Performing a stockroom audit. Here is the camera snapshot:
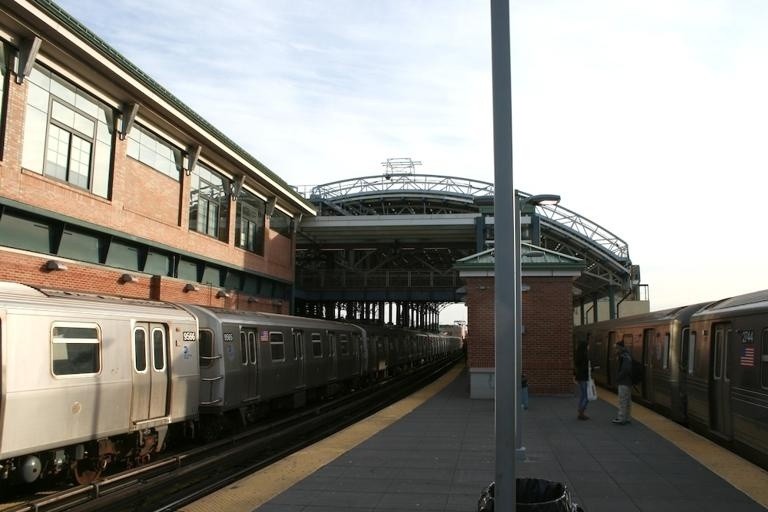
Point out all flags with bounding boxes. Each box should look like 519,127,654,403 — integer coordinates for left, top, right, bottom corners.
260,330,270,342
739,343,757,368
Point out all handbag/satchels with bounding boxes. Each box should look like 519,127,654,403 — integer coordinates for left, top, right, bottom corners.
587,379,597,402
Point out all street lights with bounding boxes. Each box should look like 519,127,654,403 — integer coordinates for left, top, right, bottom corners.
471,189,563,461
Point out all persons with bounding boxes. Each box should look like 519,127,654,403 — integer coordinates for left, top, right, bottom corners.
611,340,633,424
574,341,594,420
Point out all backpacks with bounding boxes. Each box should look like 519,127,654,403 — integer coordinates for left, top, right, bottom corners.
632,360,643,385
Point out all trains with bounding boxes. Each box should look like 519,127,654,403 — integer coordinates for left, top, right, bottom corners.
573,289,768,469
0,281,465,489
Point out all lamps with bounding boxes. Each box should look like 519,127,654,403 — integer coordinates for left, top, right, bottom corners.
248,297,258,302
186,283,200,292
457,287,467,294
219,290,230,298
41,259,68,272
122,273,141,283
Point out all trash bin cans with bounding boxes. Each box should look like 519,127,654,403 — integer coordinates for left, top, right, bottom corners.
520,376,529,410
478,478,578,511
